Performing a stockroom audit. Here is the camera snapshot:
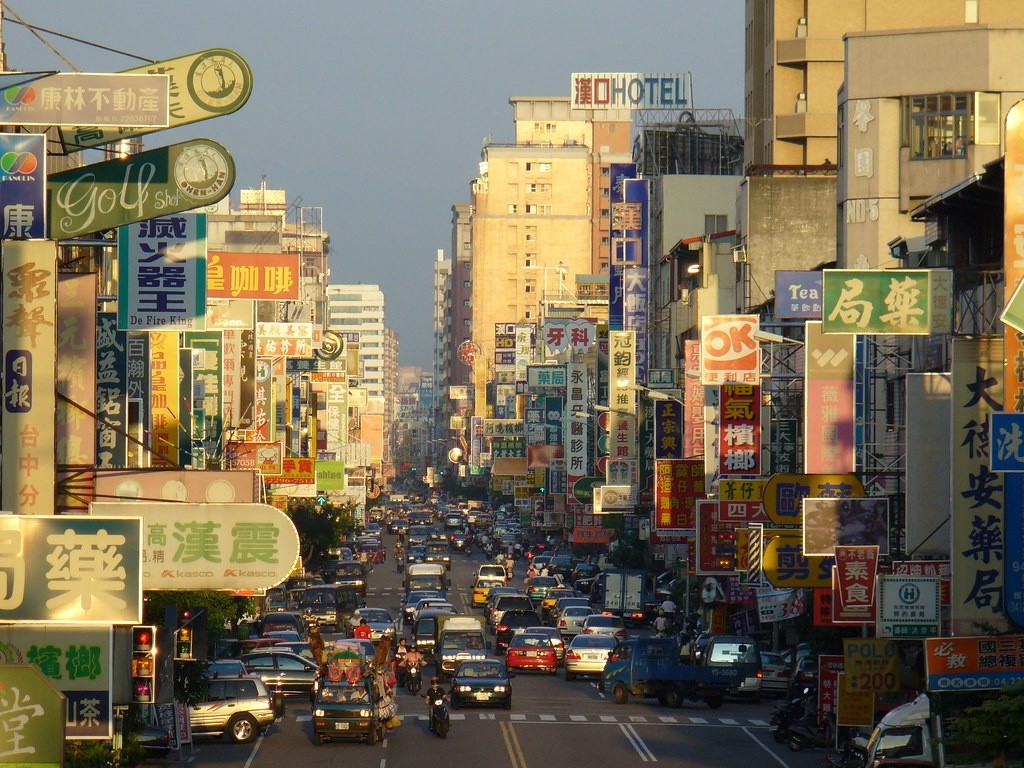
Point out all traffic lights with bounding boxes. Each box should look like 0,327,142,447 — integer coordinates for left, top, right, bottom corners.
529,486,545,493
289,495,327,506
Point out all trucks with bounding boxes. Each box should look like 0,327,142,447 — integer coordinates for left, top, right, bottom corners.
433,615,491,685
311,674,387,746
600,636,746,711
864,693,1024,768
599,567,647,630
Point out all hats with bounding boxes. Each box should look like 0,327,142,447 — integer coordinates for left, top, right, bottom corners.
659,610,664,615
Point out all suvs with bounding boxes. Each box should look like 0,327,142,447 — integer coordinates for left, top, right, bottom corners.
187,672,285,743
493,609,543,657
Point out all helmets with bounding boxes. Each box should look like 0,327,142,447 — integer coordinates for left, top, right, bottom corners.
409,643,416,648
431,677,439,684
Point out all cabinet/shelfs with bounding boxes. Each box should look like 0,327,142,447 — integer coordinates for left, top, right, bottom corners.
131,625,155,703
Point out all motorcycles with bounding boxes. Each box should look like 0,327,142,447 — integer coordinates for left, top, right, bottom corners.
420,688,453,739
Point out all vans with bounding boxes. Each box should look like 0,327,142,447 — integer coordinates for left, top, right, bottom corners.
702,635,764,705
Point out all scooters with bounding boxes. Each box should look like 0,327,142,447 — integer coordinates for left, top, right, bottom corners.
401,655,426,697
389,647,407,688
769,686,851,753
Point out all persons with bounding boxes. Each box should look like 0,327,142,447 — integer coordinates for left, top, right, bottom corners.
394,638,412,659
355,549,375,573
584,554,591,563
350,610,363,626
351,686,368,702
465,531,493,559
495,542,522,576
394,526,406,572
591,575,599,592
653,596,677,634
355,619,372,639
527,562,557,578
404,647,427,682
487,666,501,678
699,576,724,603
467,636,483,648
303,607,317,620
680,609,703,656
425,676,450,730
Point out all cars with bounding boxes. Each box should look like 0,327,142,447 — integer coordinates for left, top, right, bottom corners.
523,627,565,668
235,651,320,698
216,494,603,664
553,605,595,635
502,633,557,678
645,589,673,624
576,615,627,644
199,659,251,676
759,642,817,701
127,723,172,759
563,633,620,682
447,659,516,711
